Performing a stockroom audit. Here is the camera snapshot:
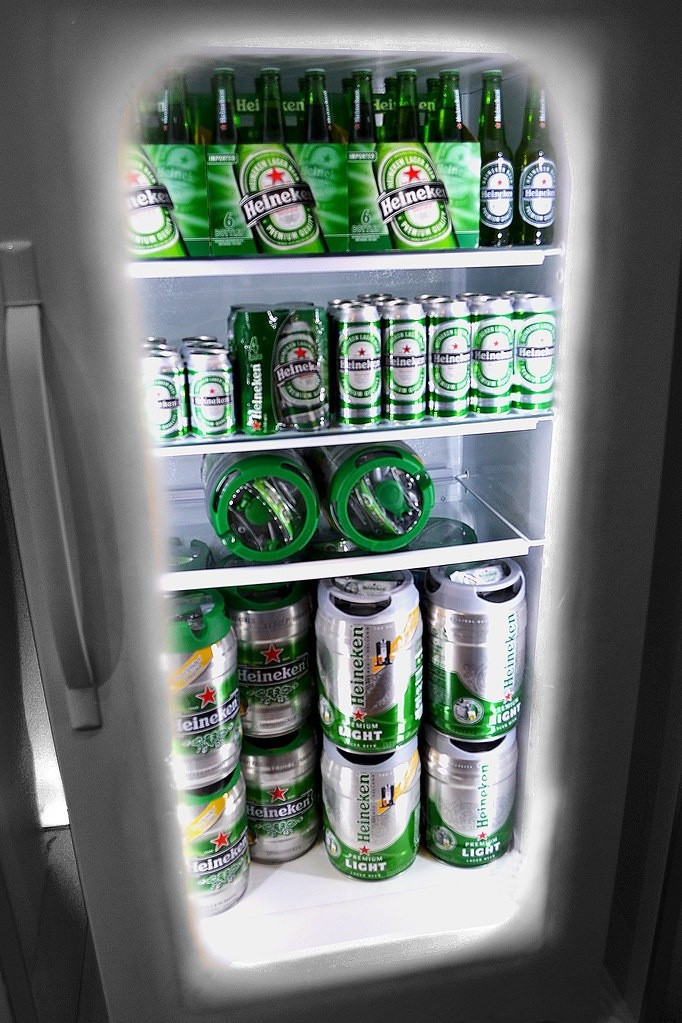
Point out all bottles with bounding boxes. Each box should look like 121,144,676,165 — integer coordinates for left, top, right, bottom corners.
372,144,460,250
127,66,463,143
232,143,330,255
478,69,516,247
515,73,556,247
125,144,190,261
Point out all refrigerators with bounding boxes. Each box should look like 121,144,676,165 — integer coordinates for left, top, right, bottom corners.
1,0,682,1023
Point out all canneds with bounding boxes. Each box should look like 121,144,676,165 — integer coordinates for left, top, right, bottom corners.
139,286,557,445
157,443,527,916
